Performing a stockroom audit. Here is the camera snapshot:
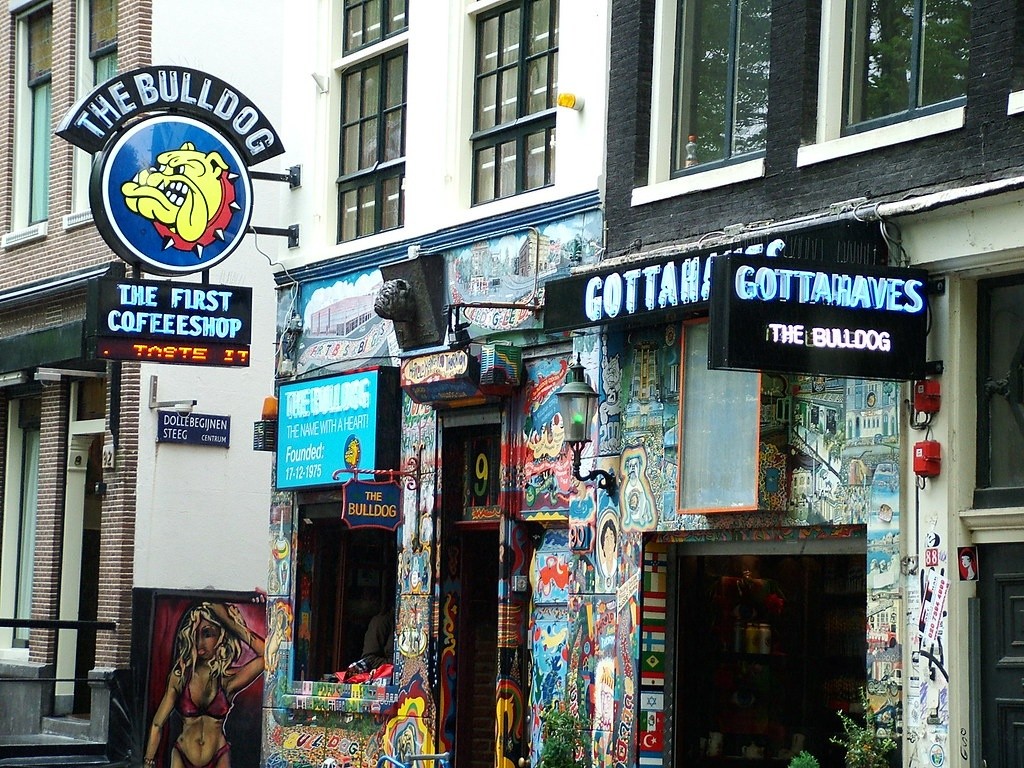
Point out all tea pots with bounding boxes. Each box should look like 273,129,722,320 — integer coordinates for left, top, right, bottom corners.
742,741,764,759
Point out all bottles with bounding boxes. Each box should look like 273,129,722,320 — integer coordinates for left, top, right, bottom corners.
745,623,759,653
760,623,771,653
730,617,744,651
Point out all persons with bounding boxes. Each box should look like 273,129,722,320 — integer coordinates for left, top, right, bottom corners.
361,586,396,670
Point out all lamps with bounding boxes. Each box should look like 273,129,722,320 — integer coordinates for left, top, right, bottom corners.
554,352,616,498
558,92,585,112
253,395,277,452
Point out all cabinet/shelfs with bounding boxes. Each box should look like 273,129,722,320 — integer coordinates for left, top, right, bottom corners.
684,555,815,768
330,531,384,672
815,556,866,768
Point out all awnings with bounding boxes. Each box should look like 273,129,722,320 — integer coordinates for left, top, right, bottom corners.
544,219,888,332
